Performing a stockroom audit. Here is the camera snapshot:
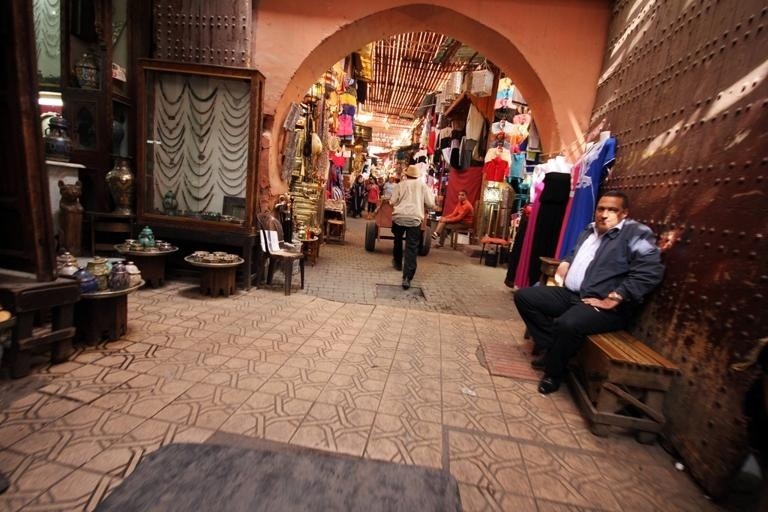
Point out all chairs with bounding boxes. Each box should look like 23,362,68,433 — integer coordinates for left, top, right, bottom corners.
451,200,482,250
479,202,512,265
256,214,307,297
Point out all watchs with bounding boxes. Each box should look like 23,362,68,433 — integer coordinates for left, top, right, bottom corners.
609,291,622,301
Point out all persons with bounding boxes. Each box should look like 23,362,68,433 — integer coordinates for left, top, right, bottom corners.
349,175,367,218
366,176,381,221
554,129,620,263
383,177,396,199
512,190,664,391
502,154,573,289
388,165,440,290
430,189,474,249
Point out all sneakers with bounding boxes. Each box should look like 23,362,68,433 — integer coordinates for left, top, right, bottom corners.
392,258,402,272
401,278,410,287
432,233,439,239
434,243,444,250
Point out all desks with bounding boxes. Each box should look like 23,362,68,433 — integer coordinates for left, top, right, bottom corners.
59,264,146,345
115,241,179,288
184,250,245,296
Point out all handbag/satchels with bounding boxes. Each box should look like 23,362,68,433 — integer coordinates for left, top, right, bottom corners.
309,116,324,157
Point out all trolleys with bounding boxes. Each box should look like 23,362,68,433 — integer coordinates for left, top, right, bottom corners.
364,196,434,255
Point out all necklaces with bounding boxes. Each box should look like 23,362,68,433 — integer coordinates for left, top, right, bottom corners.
156,78,183,213
221,84,249,197
183,82,219,212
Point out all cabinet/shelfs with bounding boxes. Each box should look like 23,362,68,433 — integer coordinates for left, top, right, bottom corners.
148,71,266,255
61,0,137,258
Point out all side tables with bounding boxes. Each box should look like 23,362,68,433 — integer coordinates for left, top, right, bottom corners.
295,232,320,266
326,220,345,246
537,255,562,288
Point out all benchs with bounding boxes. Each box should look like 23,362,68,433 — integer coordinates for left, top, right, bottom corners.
539,281,681,448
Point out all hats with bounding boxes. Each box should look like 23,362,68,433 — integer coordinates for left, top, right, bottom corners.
403,166,422,179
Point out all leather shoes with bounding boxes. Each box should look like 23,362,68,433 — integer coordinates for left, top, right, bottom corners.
537,374,561,396
529,356,547,366
